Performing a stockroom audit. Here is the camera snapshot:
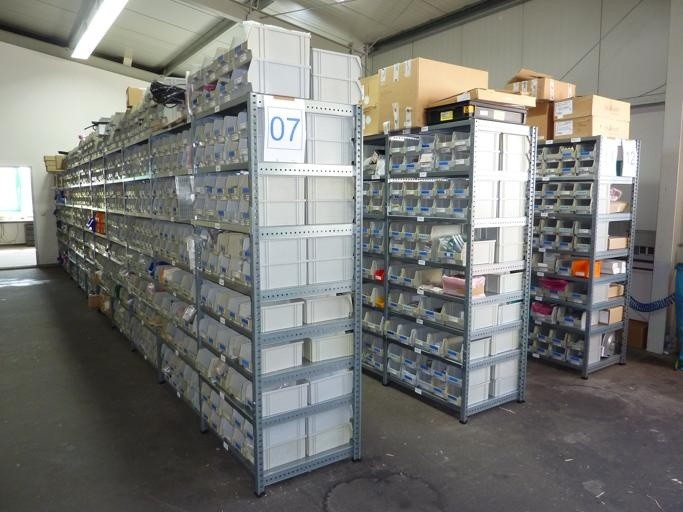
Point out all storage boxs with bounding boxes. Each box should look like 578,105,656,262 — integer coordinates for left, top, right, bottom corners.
627,319,648,349
377,56,490,134
357,74,382,136
504,68,630,140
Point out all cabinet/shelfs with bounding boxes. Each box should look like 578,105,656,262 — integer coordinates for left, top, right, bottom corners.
55,20,364,498
526,133,641,380
361,118,539,425
